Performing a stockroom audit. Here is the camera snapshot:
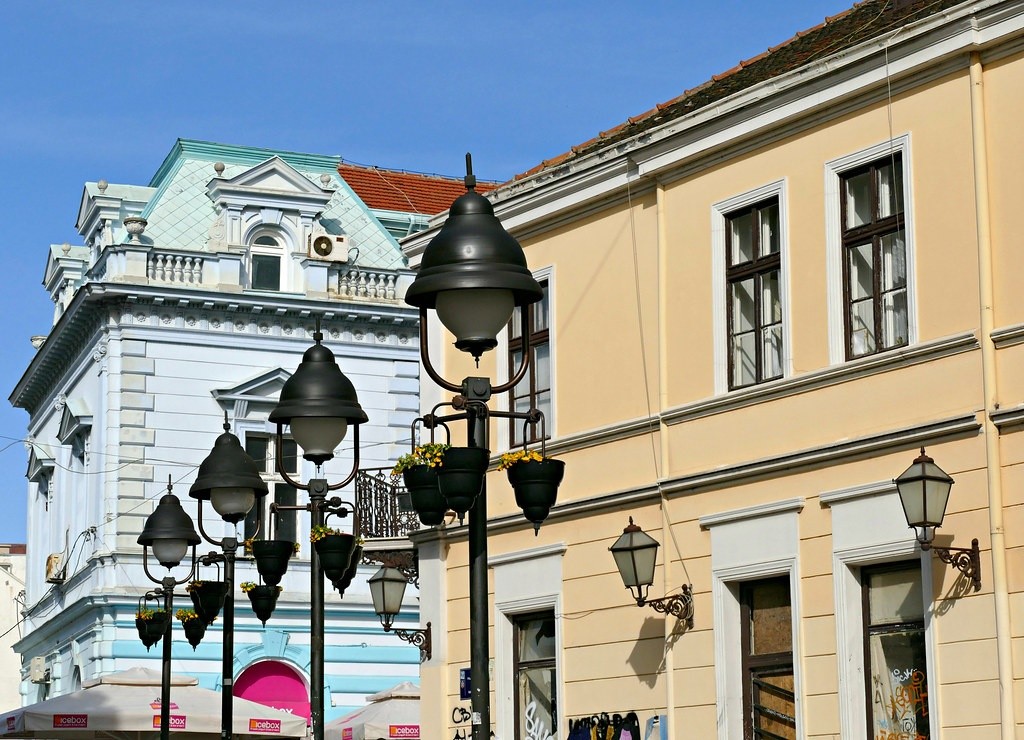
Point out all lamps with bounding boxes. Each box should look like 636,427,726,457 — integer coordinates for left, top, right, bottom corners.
609,516,697,630
893,444,982,593
366,560,432,661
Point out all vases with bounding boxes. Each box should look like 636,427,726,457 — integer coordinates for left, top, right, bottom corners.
247,585,283,628
506,457,566,537
336,545,362,599
402,465,447,527
252,541,295,597
190,590,210,631
314,534,356,591
195,581,229,627
149,611,171,647
436,446,491,527
136,618,156,652
182,618,208,652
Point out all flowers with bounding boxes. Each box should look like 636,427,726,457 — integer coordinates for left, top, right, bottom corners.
416,444,452,467
157,608,167,612
241,581,256,593
175,608,198,623
245,538,255,554
293,542,301,552
309,524,340,543
497,449,551,471
188,580,203,587
392,452,419,476
356,538,364,547
135,606,154,620
186,585,195,593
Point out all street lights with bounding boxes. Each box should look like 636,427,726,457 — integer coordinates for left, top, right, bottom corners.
190,409,285,740
136,468,210,740
400,152,569,740
268,313,379,740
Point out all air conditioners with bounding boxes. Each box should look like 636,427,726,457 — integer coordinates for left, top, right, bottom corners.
30,656,47,682
45,553,64,584
308,232,349,263
